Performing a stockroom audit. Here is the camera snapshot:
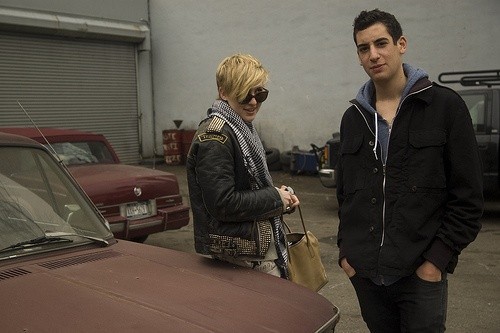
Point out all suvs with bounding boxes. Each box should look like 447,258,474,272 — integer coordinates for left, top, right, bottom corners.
320,69,500,202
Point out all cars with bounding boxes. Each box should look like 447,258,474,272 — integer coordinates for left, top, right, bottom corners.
0,126,191,243
0,131,341,333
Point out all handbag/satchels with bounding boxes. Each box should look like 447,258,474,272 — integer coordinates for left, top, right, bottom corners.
283,186,329,293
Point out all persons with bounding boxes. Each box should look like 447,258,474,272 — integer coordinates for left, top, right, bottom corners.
188,54,300,278
336,9,483,333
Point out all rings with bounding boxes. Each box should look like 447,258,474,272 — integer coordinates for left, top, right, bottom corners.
287,206,290,209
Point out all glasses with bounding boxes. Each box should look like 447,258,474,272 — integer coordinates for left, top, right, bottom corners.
237,88,269,104
